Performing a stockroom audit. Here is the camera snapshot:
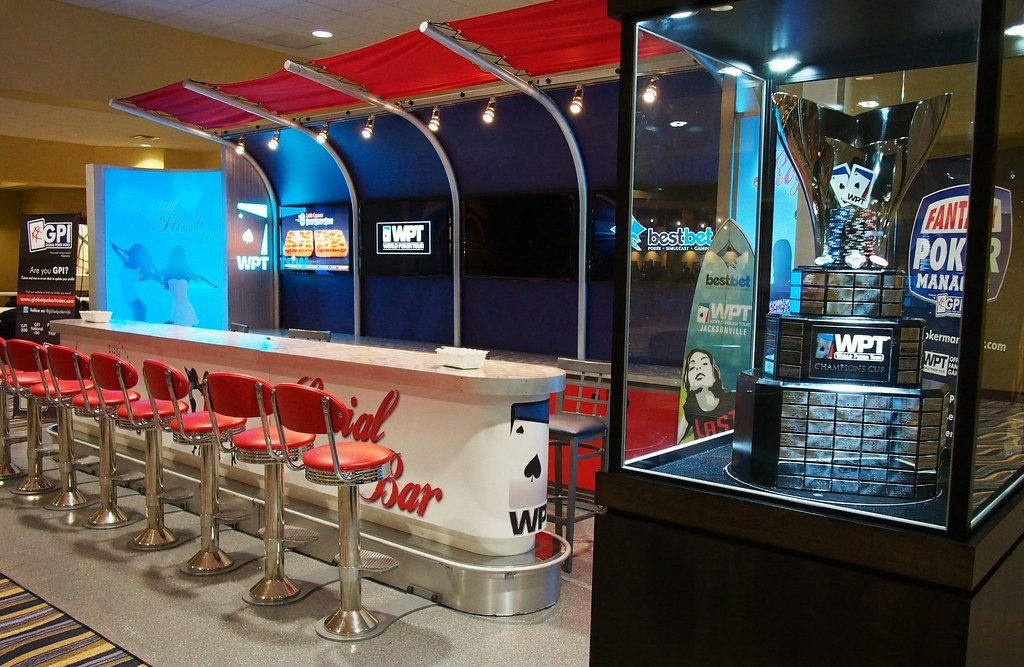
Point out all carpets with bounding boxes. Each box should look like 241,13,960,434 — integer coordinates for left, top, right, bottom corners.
0,572,149,667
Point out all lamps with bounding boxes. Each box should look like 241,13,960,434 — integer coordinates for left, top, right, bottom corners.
267,128,280,149
361,114,375,138
236,134,246,154
643,74,660,102
429,107,440,132
483,98,496,122
317,122,328,143
570,84,584,112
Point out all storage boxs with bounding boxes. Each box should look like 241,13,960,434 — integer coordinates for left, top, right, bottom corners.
435,345,489,369
79,310,113,323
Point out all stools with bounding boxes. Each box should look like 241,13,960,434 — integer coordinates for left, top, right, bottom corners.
0,338,400,642
547,357,611,573
